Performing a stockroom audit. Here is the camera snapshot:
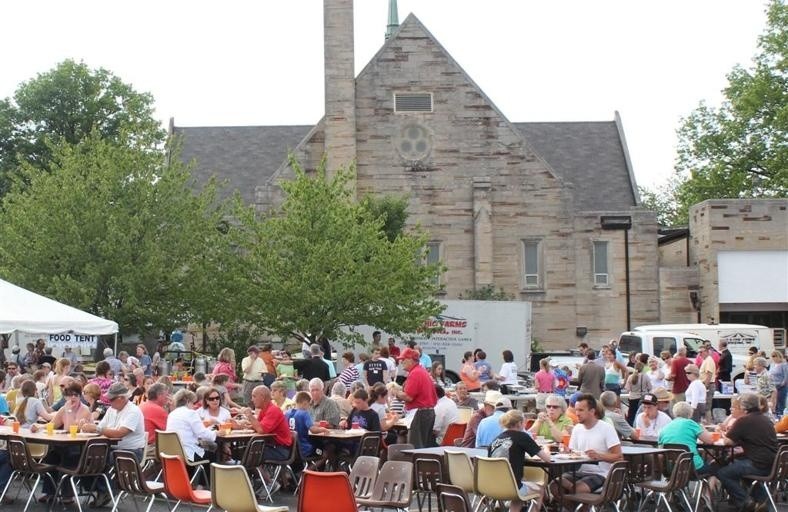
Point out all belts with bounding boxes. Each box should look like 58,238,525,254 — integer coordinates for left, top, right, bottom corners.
418,407,433,410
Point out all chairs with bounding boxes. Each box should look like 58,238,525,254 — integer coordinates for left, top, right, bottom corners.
160,452,214,512
235,430,304,495
111,449,171,512
413,453,445,512
356,460,414,512
210,463,291,512
50,438,119,512
444,451,488,511
203,435,277,505
661,444,711,511
0,436,65,512
8,442,48,502
436,485,471,512
154,430,209,493
100,431,148,503
473,455,541,512
298,470,359,512
388,445,422,512
348,456,380,512
640,452,695,512
726,447,783,512
440,423,467,445
343,432,381,468
563,461,629,512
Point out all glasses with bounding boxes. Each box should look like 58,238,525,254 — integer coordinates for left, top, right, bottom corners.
67,393,79,397
206,396,221,401
707,344,711,346
8,368,16,370
546,405,560,409
749,353,754,354
700,350,706,353
686,372,692,374
60,385,65,387
123,378,130,381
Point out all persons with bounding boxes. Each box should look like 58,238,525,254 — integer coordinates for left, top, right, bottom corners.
231,385,293,499
258,344,277,387
138,383,169,455
366,331,388,358
40,362,54,383
292,378,309,402
1,362,20,393
378,347,396,385
40,347,56,364
714,392,778,512
60,344,78,367
148,375,173,414
755,395,775,428
657,401,717,512
33,339,45,360
604,348,629,413
95,425,103,434
480,381,513,409
633,387,671,427
196,389,232,463
577,343,589,364
596,344,611,392
699,345,716,424
719,394,768,503
31,381,96,503
534,358,555,413
275,351,298,378
460,351,483,392
550,394,624,512
52,358,74,404
136,344,152,375
596,390,641,481
355,352,368,379
118,351,144,386
83,383,107,421
211,347,240,400
641,356,665,393
454,390,502,470
22,343,37,365
489,350,518,394
561,365,572,376
81,382,146,508
717,339,732,416
576,348,605,400
102,348,128,382
624,363,652,427
32,370,49,401
487,409,551,512
285,392,329,472
753,357,778,414
395,341,417,385
471,351,492,393
431,384,460,447
330,382,354,420
368,386,406,451
473,349,506,381
386,348,438,449
330,353,360,398
270,380,294,416
49,378,89,412
133,368,146,405
672,343,688,360
631,393,672,477
317,345,338,379
210,373,245,411
388,338,400,365
151,352,162,376
475,398,547,462
640,354,651,373
368,381,392,412
0,371,17,504
193,372,211,405
695,340,720,420
5,345,28,372
324,339,338,361
771,350,788,420
166,389,218,490
270,343,330,380
241,345,269,409
172,357,187,371
684,364,707,422
363,347,388,393
430,361,448,388
307,377,340,440
325,389,381,475
124,373,142,406
13,381,57,430
453,380,478,412
757,350,766,358
414,345,432,373
627,352,637,368
67,365,89,382
136,377,154,405
610,340,625,365
528,396,574,443
549,360,569,397
744,347,759,384
347,381,364,408
665,345,694,420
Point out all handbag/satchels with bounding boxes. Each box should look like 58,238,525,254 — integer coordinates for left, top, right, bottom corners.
638,374,645,405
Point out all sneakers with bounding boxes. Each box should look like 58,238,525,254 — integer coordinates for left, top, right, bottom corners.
255,480,281,499
699,494,712,511
0,496,14,506
39,495,58,502
59,497,75,504
88,491,116,508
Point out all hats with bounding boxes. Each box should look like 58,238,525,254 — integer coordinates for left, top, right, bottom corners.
104,348,113,356
649,387,674,401
549,360,559,366
641,393,657,406
396,348,420,360
12,345,20,352
103,383,128,400
495,398,512,408
248,345,262,353
484,391,502,406
41,362,51,369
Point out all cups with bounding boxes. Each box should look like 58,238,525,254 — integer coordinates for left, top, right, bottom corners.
536,435,546,446
635,429,640,438
219,424,226,434
12,422,20,434
561,436,571,448
713,432,722,440
188,376,193,382
225,423,232,435
181,376,187,382
70,425,77,439
47,423,54,436
352,422,359,429
319,420,327,432
173,376,177,381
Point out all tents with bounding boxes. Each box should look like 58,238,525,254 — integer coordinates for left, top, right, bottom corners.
0,278,120,358
